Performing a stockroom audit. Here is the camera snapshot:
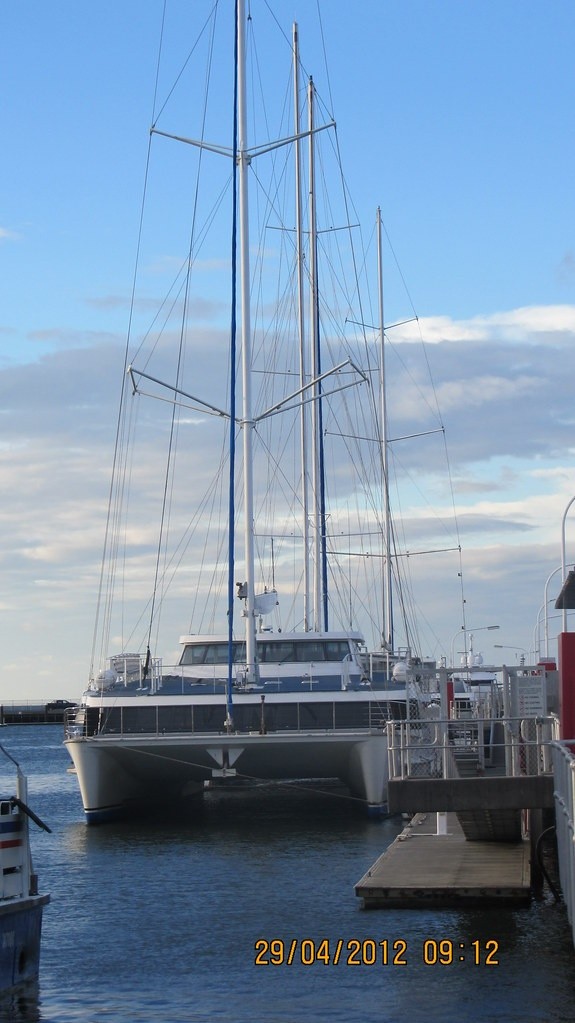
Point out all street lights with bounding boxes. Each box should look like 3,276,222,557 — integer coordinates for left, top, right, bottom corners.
495,644,530,666
450,625,501,670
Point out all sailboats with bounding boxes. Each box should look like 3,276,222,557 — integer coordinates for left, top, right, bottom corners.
58,2,447,831
